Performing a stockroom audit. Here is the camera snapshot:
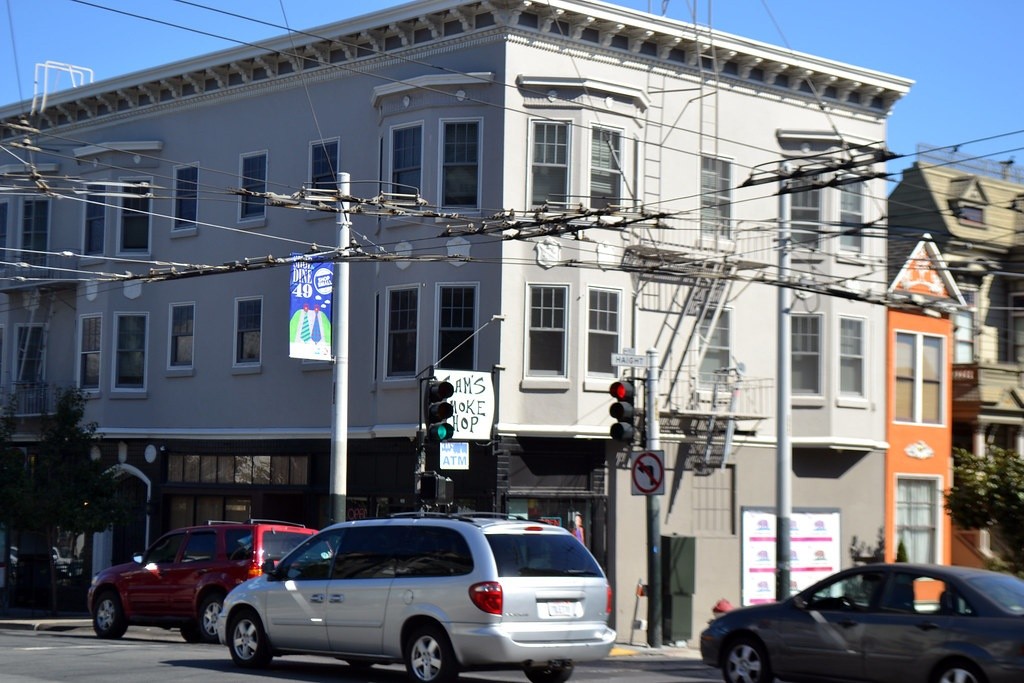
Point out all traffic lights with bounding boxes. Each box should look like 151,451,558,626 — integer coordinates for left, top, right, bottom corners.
423,474,453,506
424,376,458,444
609,377,636,443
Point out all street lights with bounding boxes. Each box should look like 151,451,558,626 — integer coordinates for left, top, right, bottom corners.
123,464,153,551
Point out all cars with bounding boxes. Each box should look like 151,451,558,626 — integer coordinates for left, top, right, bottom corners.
699,559,1024,682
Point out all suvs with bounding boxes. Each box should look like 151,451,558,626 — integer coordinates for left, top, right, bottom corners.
85,516,318,647
215,511,618,682
